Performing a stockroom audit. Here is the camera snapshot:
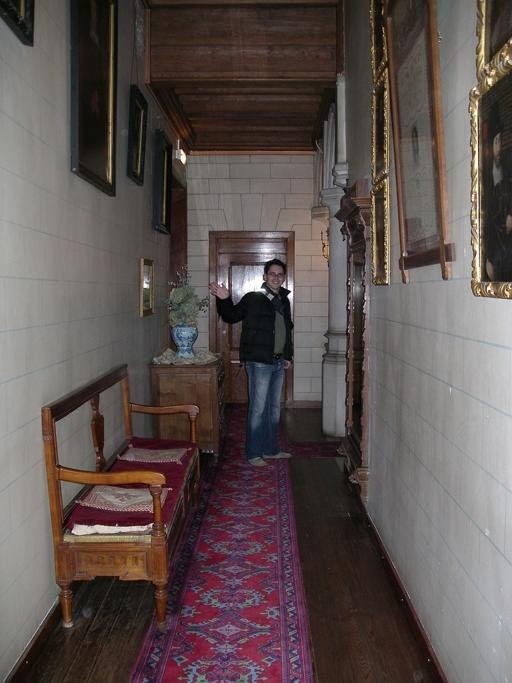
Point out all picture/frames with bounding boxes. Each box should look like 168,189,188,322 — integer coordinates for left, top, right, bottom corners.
389,2,457,290
70,0,118,197
0,0,34,46
154,131,173,234
127,83,148,186
471,4,511,291
140,258,155,318
370,4,388,288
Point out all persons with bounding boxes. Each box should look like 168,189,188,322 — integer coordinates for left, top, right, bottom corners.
481,101,512,282
208,256,295,468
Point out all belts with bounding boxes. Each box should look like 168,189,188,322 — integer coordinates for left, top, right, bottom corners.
272,352,284,360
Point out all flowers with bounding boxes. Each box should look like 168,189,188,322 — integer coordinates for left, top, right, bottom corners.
166,267,208,326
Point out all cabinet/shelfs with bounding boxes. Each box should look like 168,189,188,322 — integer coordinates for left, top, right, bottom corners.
146,347,226,467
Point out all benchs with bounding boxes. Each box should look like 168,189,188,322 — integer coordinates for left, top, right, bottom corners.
41,361,199,631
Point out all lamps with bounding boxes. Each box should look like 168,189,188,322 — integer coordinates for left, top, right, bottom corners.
171,326,198,358
